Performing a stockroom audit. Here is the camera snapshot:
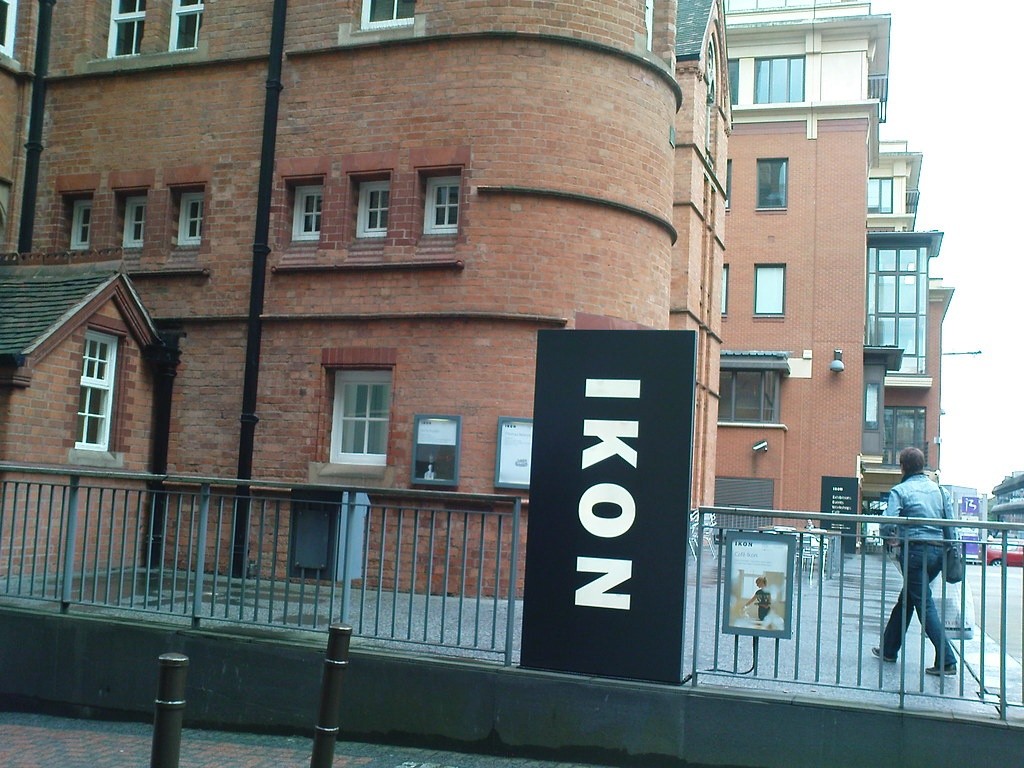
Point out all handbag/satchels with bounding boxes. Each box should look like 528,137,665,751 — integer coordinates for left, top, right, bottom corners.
947,547,962,584
920,571,974,639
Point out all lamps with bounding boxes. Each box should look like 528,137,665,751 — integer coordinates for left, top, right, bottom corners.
830,350,844,372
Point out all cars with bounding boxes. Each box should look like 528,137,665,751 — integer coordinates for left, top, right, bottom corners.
978,537,1024,569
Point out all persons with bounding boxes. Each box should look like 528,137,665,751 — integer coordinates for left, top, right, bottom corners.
870,447,957,675
804,519,816,529
746,577,771,621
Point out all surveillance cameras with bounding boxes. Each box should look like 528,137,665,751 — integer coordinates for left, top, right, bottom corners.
752,441,767,453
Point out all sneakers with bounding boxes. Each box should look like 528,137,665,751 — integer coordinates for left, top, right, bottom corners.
926,663,956,675
872,648,897,662
